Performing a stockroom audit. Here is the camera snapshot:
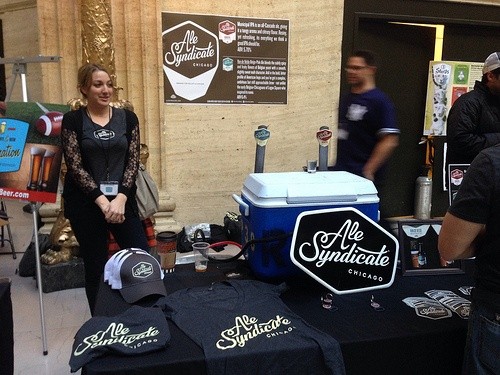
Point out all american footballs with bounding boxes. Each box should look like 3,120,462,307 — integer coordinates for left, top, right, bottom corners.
35,111,64,137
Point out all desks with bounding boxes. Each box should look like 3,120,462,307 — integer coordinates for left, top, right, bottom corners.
78,250,476,374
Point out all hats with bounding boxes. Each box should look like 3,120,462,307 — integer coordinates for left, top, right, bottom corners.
104,247,167,303
484,52,500,74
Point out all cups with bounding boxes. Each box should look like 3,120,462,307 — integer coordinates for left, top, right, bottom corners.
42,151,56,187
27,147,46,188
156,231,177,273
192,243,209,272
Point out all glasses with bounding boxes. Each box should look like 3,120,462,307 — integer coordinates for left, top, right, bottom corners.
344,65,367,70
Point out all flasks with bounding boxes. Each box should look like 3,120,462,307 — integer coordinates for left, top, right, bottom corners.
414,177,432,220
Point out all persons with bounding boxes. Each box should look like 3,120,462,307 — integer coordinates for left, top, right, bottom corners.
60,62,151,317
438,143,500,375
446,50,500,168
332,50,401,181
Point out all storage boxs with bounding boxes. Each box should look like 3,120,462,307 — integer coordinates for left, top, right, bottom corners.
232,171,381,276
